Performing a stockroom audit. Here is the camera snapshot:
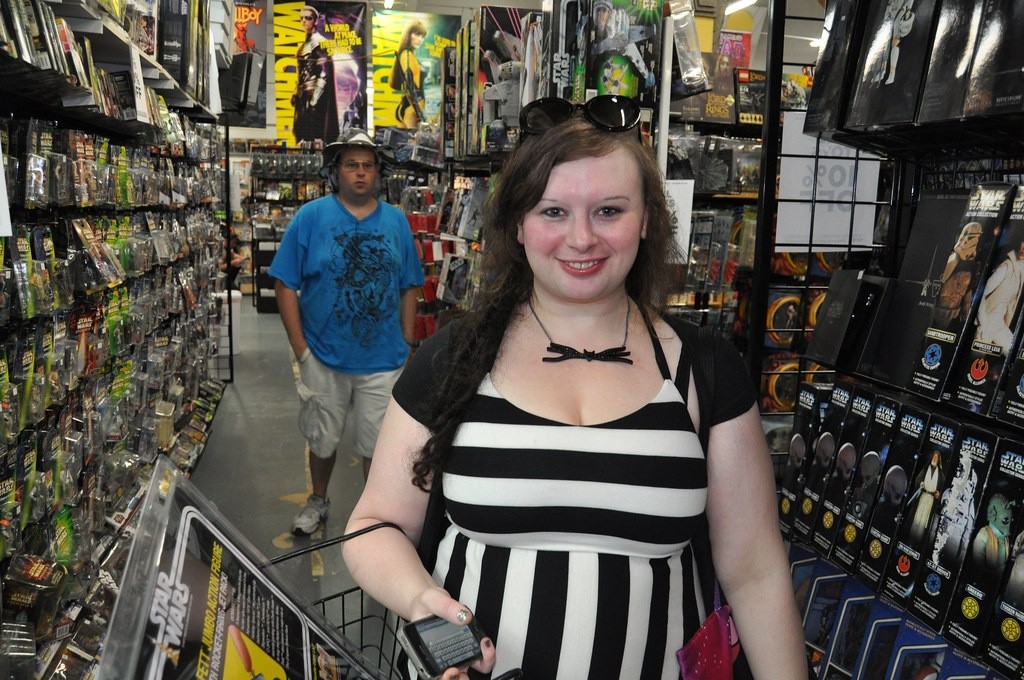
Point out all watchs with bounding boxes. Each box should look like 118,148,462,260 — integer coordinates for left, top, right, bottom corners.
407,339,421,348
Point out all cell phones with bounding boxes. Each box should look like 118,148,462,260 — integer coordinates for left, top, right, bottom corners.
395,605,486,680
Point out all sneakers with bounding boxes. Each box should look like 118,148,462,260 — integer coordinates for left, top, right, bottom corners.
290,493,328,536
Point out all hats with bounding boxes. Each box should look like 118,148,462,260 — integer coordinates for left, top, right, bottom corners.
322,127,399,193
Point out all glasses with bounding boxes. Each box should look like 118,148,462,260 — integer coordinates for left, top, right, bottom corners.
300,16,313,21
335,160,381,173
519,93,643,144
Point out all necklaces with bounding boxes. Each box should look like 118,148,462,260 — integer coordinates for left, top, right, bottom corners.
527,292,634,365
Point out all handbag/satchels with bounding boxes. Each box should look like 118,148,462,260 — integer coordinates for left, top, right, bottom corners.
674,324,756,680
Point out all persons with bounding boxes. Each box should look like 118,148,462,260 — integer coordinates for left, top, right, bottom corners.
714,53,732,92
388,20,428,129
973,236,1024,357
591,0,613,46
341,94,808,680
267,129,426,535
290,6,341,144
905,450,947,540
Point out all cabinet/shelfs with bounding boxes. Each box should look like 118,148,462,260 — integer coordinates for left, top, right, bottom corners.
1,2,1024,680
